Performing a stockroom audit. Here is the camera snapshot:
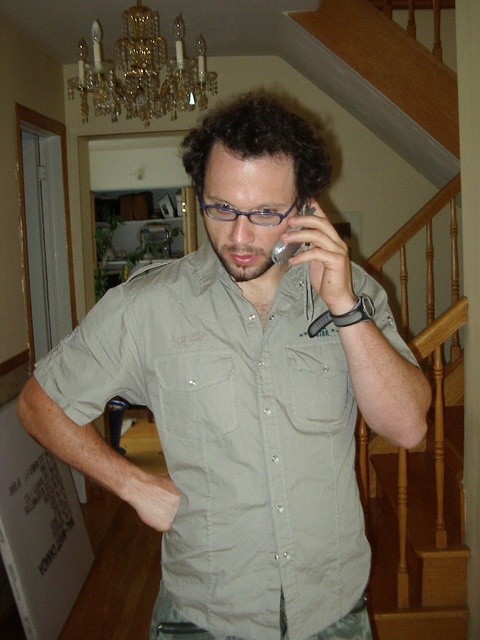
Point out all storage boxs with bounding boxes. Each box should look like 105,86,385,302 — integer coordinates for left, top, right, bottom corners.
176,194,182,216
120,195,133,219
141,231,170,257
133,192,153,219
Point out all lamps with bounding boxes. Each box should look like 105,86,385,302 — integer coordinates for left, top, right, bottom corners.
67,0,219,127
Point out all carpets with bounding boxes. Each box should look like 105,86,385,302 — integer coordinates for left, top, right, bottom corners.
121,418,136,436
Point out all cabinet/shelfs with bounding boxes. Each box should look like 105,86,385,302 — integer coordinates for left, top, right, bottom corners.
79,130,198,326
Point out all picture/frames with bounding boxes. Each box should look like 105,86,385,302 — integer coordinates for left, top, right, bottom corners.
326,212,361,266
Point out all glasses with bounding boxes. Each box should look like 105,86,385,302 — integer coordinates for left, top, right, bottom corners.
198,189,301,227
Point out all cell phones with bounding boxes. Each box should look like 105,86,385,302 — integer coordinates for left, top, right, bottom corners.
270,202,316,266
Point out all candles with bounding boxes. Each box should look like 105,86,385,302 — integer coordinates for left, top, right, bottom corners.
76,37,89,82
174,12,185,67
91,19,103,69
196,34,207,82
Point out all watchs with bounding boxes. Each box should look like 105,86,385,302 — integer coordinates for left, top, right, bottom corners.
307,294,375,339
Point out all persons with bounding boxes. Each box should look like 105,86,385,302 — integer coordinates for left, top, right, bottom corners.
15,88,432,638
